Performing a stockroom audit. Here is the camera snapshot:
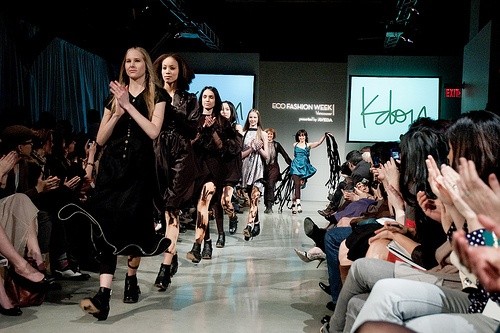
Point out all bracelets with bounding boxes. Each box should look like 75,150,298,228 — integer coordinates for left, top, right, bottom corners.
87,163,94,166
367,195,372,198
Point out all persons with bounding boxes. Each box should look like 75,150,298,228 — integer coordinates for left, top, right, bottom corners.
0,81,500,333
152,53,201,289
78,47,172,320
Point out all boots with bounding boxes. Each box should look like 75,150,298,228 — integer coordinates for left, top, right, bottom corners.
264,202,268,212
318,202,338,217
268,202,273,212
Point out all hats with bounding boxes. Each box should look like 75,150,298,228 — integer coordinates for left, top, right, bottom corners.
1,125,35,143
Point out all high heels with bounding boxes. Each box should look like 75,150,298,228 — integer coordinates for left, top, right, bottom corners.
201,239,212,258
124,273,139,302
291,204,297,214
294,245,326,268
79,287,111,321
186,243,201,264
297,203,302,213
153,264,172,290
170,251,178,277
229,215,238,234
243,225,252,241
216,232,225,247
252,221,260,237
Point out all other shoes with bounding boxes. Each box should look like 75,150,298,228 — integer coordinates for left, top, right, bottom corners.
318,279,335,333
12,270,58,291
55,265,91,280
303,216,327,252
0,304,23,316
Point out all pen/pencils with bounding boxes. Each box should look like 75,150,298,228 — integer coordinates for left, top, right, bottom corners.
387,224,399,227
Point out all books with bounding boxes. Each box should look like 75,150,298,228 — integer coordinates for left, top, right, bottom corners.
386,240,426,273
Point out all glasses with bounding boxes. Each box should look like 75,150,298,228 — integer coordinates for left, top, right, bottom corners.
19,141,32,145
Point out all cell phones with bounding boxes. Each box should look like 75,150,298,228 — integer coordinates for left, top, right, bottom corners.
390,148,400,167
